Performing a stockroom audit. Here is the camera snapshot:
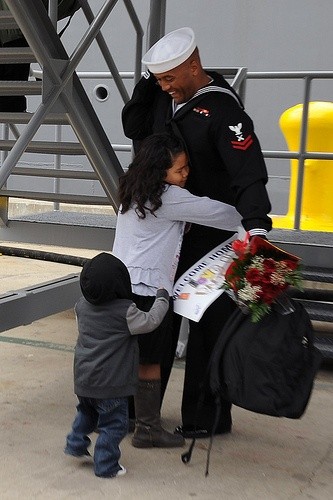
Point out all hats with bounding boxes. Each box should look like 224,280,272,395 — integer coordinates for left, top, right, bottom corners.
141,27,196,73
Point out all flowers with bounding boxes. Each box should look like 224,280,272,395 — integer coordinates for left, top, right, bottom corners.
216,236,305,321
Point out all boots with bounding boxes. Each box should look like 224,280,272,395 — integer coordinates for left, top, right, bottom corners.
132,379,185,448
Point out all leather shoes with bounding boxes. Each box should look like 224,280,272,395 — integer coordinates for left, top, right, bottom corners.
175,424,210,437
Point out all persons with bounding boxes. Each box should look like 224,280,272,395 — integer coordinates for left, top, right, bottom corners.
121,24,273,438
111,130,243,449
65,253,170,479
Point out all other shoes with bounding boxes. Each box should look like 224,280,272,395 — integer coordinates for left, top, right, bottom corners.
79,450,93,462
117,464,127,474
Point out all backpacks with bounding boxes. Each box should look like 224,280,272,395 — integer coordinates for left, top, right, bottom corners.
210,294,323,419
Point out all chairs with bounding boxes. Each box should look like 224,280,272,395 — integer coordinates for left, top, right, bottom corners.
270,101,333,233
